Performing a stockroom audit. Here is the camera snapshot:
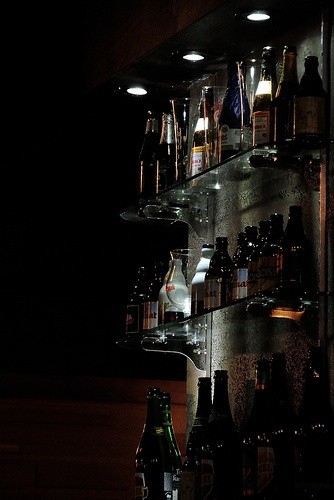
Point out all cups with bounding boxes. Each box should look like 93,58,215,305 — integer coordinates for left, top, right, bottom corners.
202,85,228,173
237,57,262,151
168,96,199,187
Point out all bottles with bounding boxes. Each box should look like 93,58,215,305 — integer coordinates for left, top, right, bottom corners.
137,111,162,216
220,60,251,182
134,345,334,499
124,205,313,335
249,43,282,171
190,85,216,196
272,44,303,168
154,110,175,202
292,56,327,149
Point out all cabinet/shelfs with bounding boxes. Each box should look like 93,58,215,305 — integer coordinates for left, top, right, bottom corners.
85,0,334,500
